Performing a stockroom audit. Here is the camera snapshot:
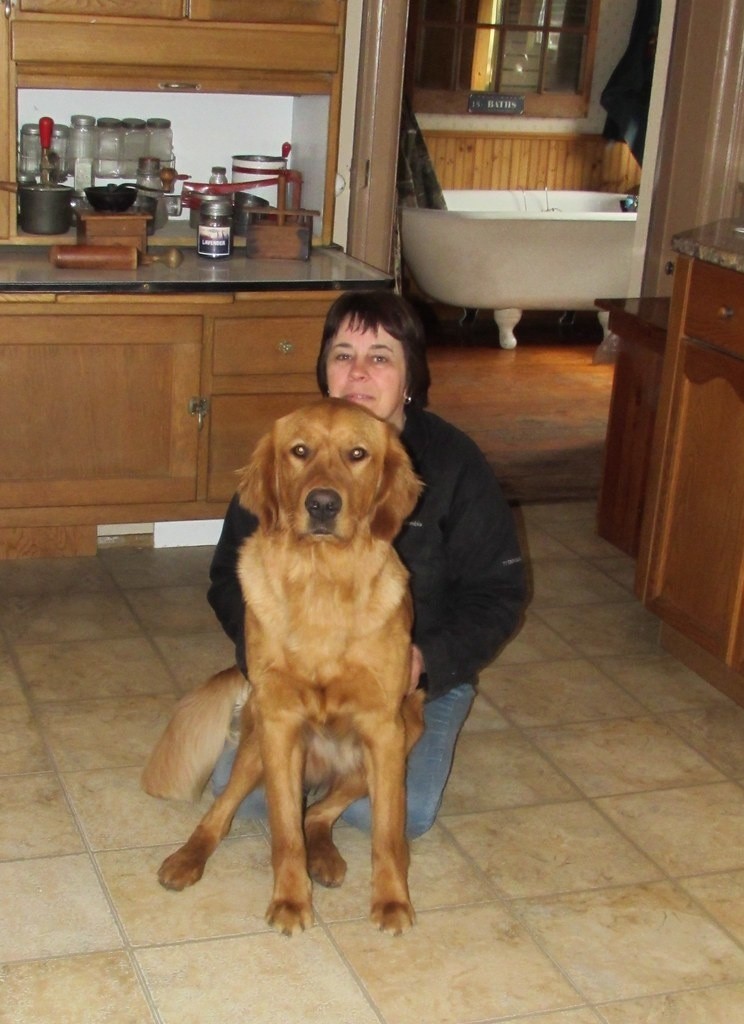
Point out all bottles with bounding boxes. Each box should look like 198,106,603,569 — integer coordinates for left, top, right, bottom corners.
19,124,43,176
45,124,69,175
196,199,233,259
95,118,122,180
145,118,172,175
135,156,170,230
119,118,148,179
68,115,99,178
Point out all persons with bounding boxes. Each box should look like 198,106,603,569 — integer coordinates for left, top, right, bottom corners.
207,290,527,839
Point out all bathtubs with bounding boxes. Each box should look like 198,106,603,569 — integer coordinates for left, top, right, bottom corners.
396,180,649,349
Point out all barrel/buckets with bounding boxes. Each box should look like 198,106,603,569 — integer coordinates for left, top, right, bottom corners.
18,184,74,235
231,155,288,241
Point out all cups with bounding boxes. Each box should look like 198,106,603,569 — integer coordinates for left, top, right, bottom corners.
209,167,230,199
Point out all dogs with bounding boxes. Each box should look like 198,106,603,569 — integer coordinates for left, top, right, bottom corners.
137,399,426,938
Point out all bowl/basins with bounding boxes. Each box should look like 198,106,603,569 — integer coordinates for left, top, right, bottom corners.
83,186,137,213
